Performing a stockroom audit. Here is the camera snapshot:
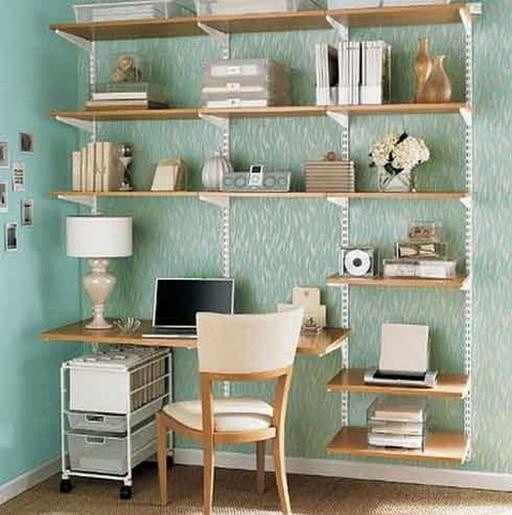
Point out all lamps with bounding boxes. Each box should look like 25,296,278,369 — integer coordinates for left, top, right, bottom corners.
63,214,134,330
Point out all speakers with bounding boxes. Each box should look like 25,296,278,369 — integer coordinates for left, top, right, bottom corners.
223,171,291,193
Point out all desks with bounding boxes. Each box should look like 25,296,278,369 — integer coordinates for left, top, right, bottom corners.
39,317,353,502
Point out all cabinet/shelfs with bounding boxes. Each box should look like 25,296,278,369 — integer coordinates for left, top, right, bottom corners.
46,4,472,464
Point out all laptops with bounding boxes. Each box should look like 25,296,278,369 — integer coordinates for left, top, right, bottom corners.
141,277,235,338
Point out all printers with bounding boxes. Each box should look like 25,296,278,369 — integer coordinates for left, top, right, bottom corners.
364,336,439,388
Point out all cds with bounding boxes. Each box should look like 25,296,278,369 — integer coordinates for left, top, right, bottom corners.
344,250,370,277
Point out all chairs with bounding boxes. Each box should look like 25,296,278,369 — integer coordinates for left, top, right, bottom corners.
153,304,310,514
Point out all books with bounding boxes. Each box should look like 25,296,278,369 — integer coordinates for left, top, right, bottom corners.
131,359,165,411
91,4,165,21
85,92,169,111
314,37,391,104
71,141,124,192
211,0,294,17
